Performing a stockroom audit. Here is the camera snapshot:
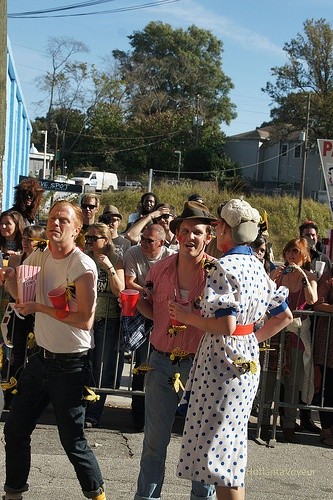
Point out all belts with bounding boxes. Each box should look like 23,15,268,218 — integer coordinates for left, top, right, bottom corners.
232,324,254,335
154,348,196,361
41,347,87,360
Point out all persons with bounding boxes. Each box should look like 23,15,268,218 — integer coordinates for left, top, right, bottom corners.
117,200,220,500
0,199,107,500
168,199,293,500
0,177,333,446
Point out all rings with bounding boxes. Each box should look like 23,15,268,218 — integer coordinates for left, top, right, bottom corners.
169,310,172,313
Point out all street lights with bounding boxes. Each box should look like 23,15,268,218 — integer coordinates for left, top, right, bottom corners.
36,130,47,179
174,150,181,181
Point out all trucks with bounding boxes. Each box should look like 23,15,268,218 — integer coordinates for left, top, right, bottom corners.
71,171,118,192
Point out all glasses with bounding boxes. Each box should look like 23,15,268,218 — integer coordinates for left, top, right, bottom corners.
84,235,105,241
105,218,116,224
141,235,155,243
284,249,298,255
81,204,96,209
155,214,171,222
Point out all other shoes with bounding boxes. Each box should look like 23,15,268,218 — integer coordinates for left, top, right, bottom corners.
258,427,270,441
283,428,299,443
321,435,333,448
299,419,320,433
84,418,98,428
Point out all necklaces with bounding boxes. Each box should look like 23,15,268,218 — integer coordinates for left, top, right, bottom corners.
288,272,301,304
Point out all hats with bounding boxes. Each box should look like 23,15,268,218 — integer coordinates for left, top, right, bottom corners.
169,201,218,234
99,205,123,220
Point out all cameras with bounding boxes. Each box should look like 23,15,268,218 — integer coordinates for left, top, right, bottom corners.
282,266,295,275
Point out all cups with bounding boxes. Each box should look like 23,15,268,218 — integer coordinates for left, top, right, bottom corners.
47,287,70,312
120,289,139,317
170,298,188,327
316,261,326,275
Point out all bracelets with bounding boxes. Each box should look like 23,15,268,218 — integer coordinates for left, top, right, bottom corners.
301,276,310,287
106,265,113,272
108,271,116,276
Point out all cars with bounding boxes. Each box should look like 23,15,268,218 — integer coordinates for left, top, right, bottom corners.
54,175,76,185
118,181,142,191
